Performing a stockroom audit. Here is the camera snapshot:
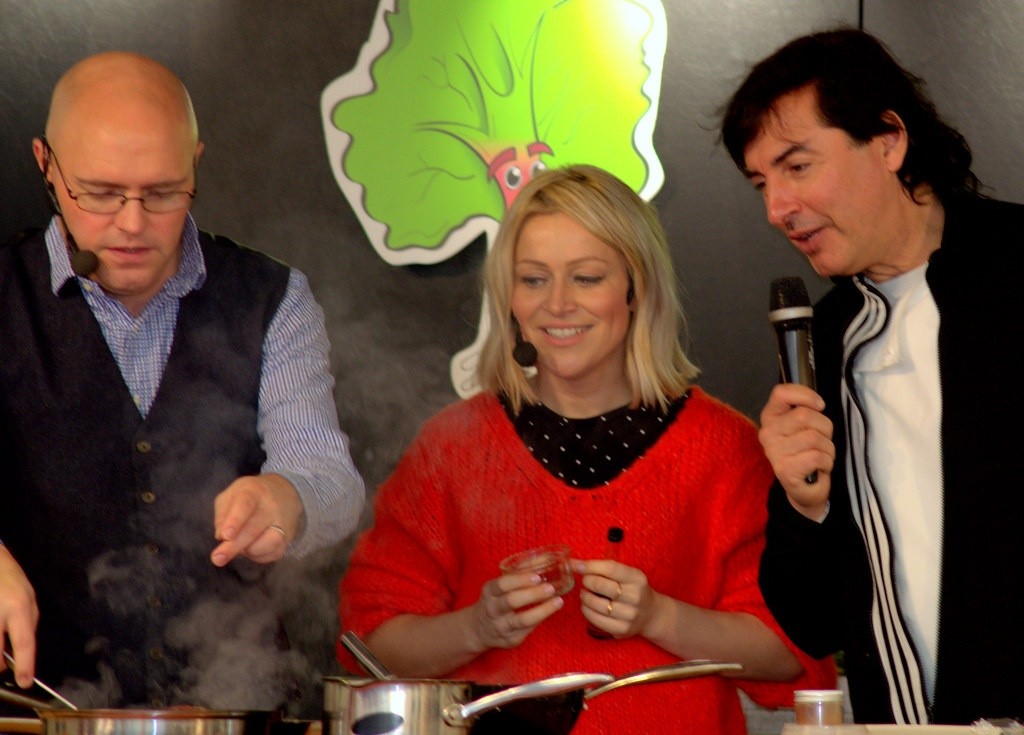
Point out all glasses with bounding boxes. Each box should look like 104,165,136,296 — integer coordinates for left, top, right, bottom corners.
42,136,198,215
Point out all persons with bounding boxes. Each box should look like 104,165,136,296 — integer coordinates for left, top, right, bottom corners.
333,161,838,735
714,25,1024,726
0,50,365,718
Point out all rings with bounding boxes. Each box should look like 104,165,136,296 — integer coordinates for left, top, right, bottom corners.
612,583,623,600
270,526,287,537
606,600,614,616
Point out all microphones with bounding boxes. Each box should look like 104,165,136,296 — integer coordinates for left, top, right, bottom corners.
510,309,538,366
767,274,818,484
42,177,98,280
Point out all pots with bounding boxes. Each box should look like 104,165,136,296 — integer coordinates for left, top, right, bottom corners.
1,659,747,735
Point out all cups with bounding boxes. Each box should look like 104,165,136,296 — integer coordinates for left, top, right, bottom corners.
500,545,574,594
